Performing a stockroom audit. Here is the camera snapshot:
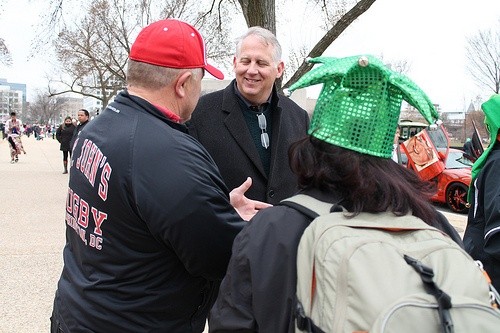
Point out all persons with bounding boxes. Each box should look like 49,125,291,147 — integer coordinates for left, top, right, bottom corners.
390,122,401,165
0,109,89,175
50,20,274,333
462,93,500,310
208,54,500,333
185,26,311,205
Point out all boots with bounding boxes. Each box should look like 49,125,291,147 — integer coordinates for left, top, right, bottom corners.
62,161,68,174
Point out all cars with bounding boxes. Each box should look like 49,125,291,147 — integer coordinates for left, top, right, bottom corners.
406,148,477,214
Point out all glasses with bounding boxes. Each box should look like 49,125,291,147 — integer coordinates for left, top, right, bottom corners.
255,113,270,150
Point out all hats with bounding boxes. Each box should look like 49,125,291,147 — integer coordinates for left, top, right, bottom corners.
127,17,225,82
464,93,500,209
285,55,444,159
